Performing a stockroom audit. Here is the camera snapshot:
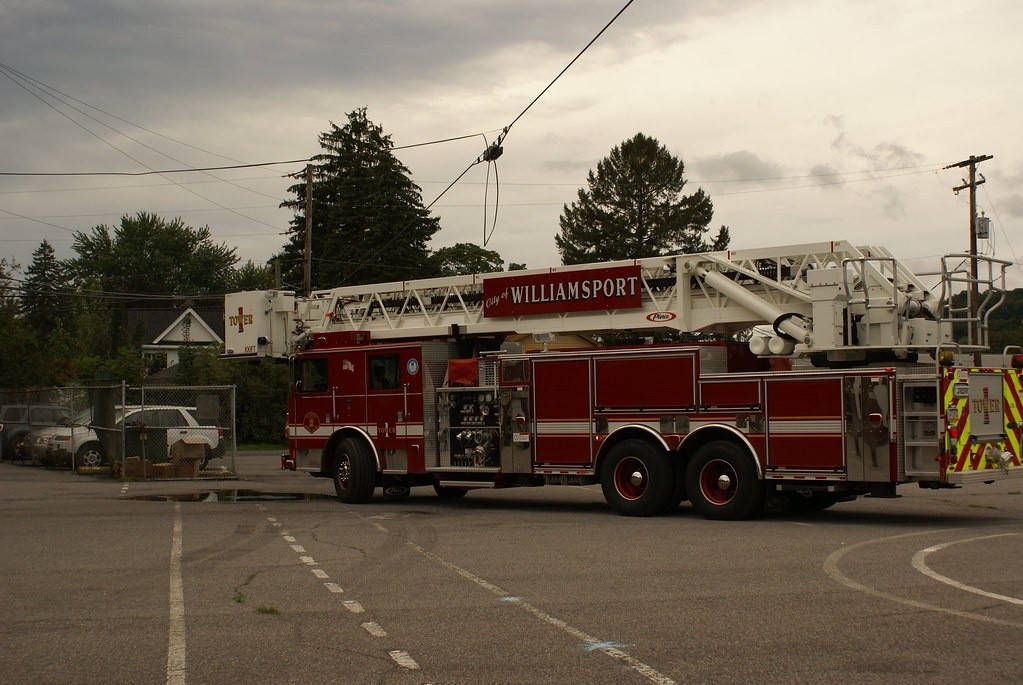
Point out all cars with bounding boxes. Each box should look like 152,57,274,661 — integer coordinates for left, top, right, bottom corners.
21,405,225,470
0,405,79,462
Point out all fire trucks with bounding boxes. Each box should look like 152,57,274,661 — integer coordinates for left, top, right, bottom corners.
216,240,1023,517
